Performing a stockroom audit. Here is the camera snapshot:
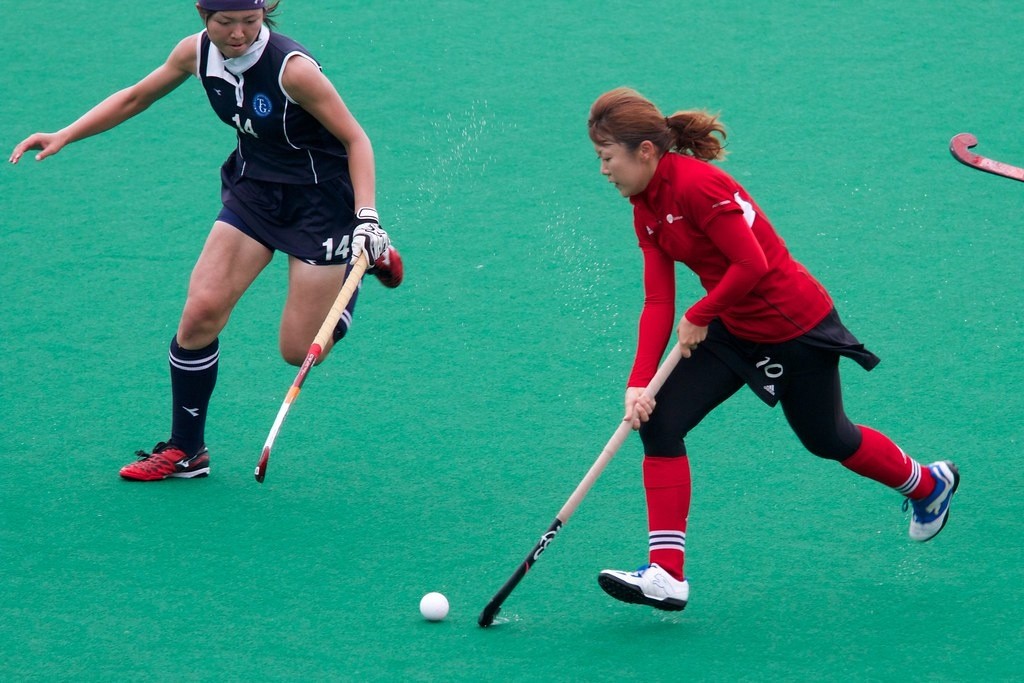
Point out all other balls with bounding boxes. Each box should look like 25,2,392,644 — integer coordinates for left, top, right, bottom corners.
419,592,449,620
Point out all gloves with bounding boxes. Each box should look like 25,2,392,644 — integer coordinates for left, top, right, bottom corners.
349,207,391,268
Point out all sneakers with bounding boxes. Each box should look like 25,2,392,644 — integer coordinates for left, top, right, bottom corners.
598,562,691,612
119,439,212,481
903,461,960,542
365,243,403,288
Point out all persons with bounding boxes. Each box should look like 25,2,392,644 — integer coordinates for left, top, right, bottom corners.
588,87,961,612
10,1,403,481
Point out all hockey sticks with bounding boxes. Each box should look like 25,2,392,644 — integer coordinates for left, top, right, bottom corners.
254,250,368,482
950,131,1022,181
476,337,684,626
